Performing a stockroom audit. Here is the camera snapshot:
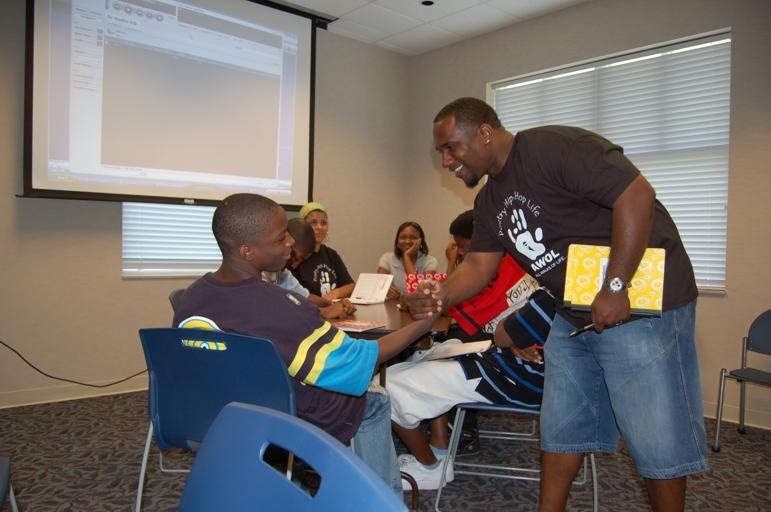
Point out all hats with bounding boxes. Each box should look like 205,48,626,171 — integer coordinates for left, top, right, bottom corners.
298,202,328,220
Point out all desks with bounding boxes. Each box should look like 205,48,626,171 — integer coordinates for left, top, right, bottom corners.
321,297,452,388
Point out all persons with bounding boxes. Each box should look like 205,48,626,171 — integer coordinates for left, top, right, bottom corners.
168,192,445,503
377,222,439,301
292,201,356,307
262,218,357,319
408,95,713,511
372,284,558,491
426,209,543,458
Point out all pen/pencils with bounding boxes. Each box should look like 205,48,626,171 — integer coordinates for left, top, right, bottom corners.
570,323,596,337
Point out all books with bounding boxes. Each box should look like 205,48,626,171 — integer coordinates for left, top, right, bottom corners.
333,319,385,333
563,243,667,319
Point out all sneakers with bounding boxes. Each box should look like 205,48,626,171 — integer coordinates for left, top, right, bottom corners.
396,432,482,492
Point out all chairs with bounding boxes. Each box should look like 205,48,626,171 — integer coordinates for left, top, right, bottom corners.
435,402,600,512
0,455,21,512
134,327,355,512
713,310,771,452
177,401,410,512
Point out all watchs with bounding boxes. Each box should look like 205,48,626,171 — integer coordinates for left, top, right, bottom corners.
605,276,632,297
